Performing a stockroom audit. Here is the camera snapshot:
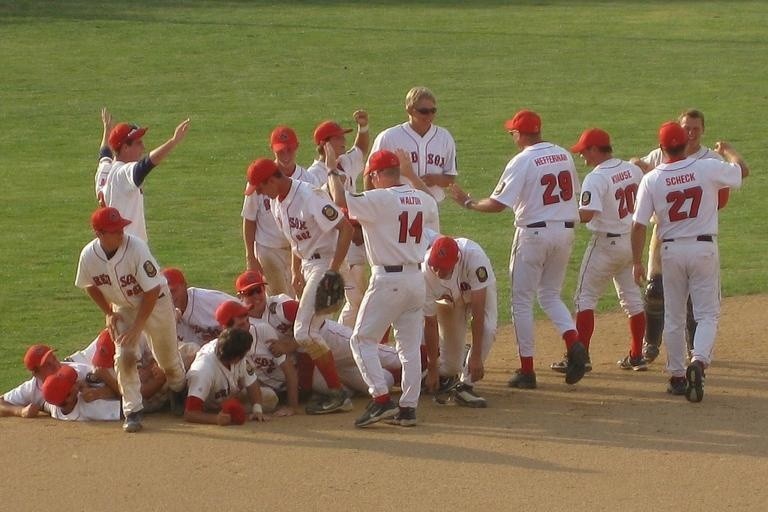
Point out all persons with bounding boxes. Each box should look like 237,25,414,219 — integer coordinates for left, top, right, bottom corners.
450,110,590,389
630,107,730,366
0,87,499,431
631,120,749,402
549,127,648,371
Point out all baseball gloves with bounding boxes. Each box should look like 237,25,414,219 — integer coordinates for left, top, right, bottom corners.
314,271,346,316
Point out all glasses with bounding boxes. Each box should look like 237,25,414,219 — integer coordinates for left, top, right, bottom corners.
241,287,263,296
415,107,438,114
506,129,522,136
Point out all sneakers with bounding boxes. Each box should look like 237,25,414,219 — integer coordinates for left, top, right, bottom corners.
122,410,144,434
686,360,706,401
306,376,488,428
666,375,687,395
168,388,188,417
508,344,659,386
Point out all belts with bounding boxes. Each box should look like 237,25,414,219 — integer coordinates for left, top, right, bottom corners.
606,232,622,239
525,222,576,229
663,236,713,242
307,252,323,259
381,264,424,272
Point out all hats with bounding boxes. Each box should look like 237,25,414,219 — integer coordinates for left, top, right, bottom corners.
161,265,186,289
92,329,115,368
427,236,459,270
214,301,255,326
234,269,268,295
90,206,132,231
569,127,610,154
245,122,401,195
41,365,78,405
23,343,58,369
504,110,542,135
108,122,149,150
656,120,687,147
